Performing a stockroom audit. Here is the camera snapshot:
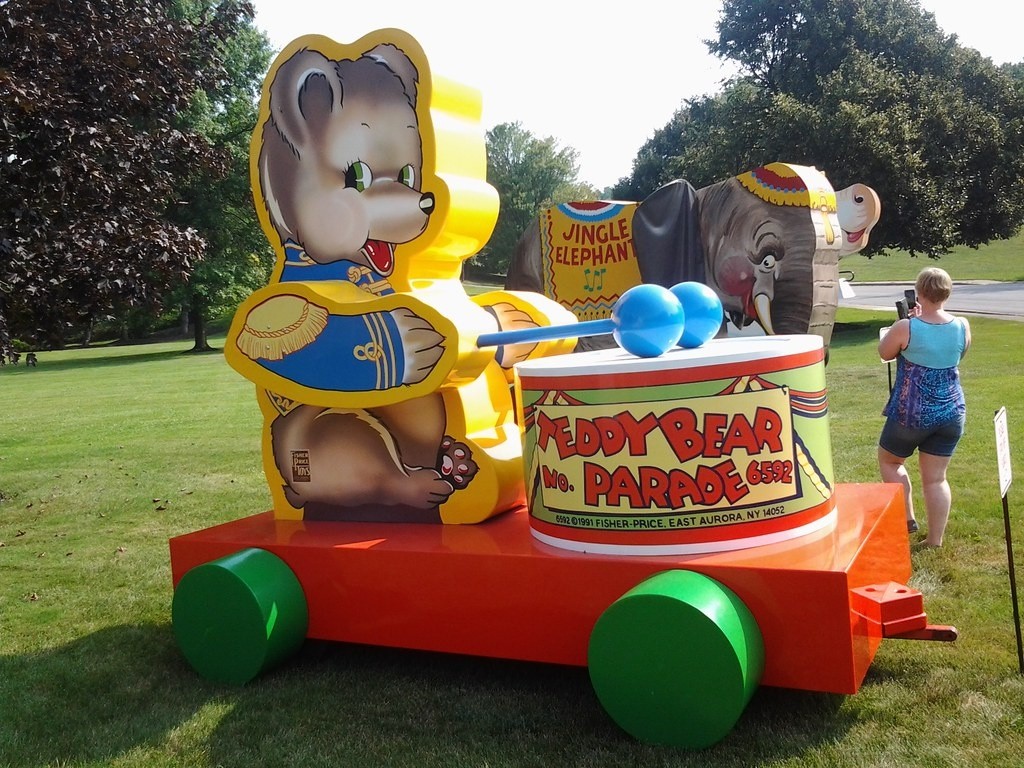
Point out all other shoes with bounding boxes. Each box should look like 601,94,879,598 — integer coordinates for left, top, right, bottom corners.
907,520,919,533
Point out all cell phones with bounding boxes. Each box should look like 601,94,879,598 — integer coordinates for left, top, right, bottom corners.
905,290,916,309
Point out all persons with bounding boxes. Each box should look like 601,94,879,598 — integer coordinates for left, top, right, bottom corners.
878,267,971,547
